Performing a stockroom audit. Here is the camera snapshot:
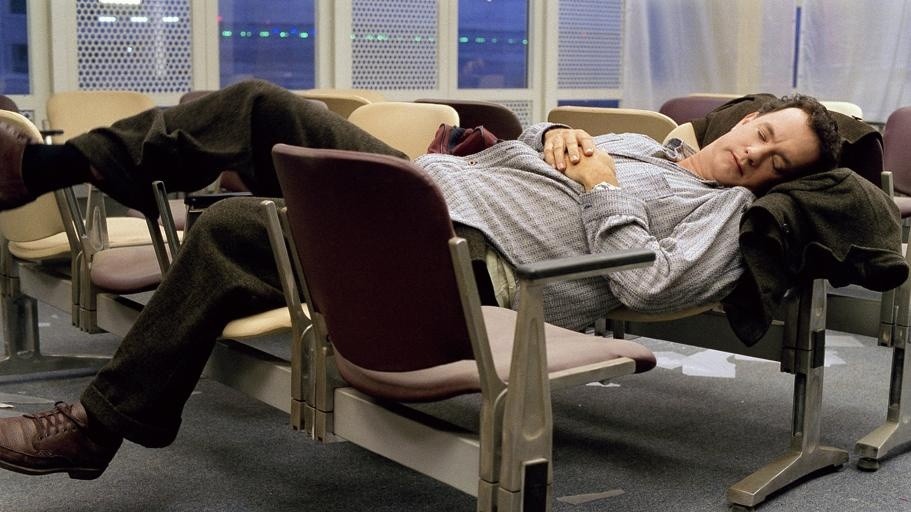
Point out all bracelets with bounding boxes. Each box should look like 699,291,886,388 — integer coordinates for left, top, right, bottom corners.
592,180,624,194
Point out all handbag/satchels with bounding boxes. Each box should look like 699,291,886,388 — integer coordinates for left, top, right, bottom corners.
426,124,497,158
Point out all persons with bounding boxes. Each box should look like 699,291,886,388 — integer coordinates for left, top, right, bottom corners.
0,77,840,482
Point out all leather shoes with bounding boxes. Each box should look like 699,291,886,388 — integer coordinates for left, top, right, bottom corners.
0,401,123,479
0,121,30,214
